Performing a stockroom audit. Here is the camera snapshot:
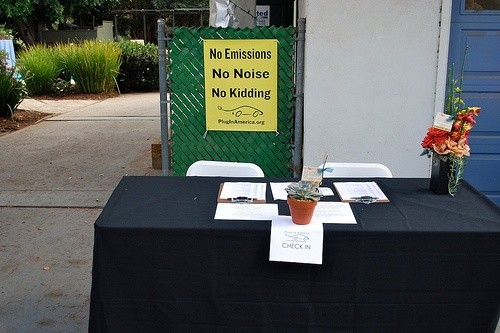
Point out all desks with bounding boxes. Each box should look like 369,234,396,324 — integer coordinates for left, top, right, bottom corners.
88,176,500,333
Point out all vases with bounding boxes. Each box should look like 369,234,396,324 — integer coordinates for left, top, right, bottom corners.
429,149,456,194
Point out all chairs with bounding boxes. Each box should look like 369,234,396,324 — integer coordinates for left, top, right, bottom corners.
185,160,264,177
318,163,394,178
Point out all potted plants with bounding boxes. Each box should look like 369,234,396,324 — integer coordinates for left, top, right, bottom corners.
285,180,324,225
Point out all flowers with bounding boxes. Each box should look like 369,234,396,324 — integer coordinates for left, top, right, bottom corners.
420,43,482,198
